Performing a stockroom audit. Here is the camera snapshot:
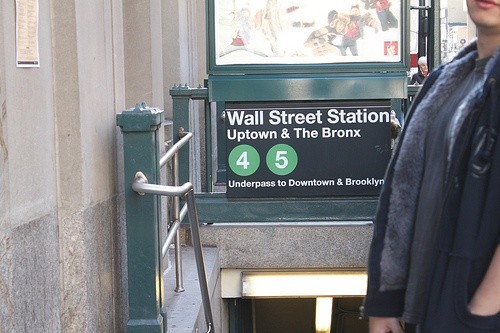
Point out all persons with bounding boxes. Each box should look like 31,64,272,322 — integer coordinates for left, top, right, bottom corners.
409,56,429,86
361,0,500,333
